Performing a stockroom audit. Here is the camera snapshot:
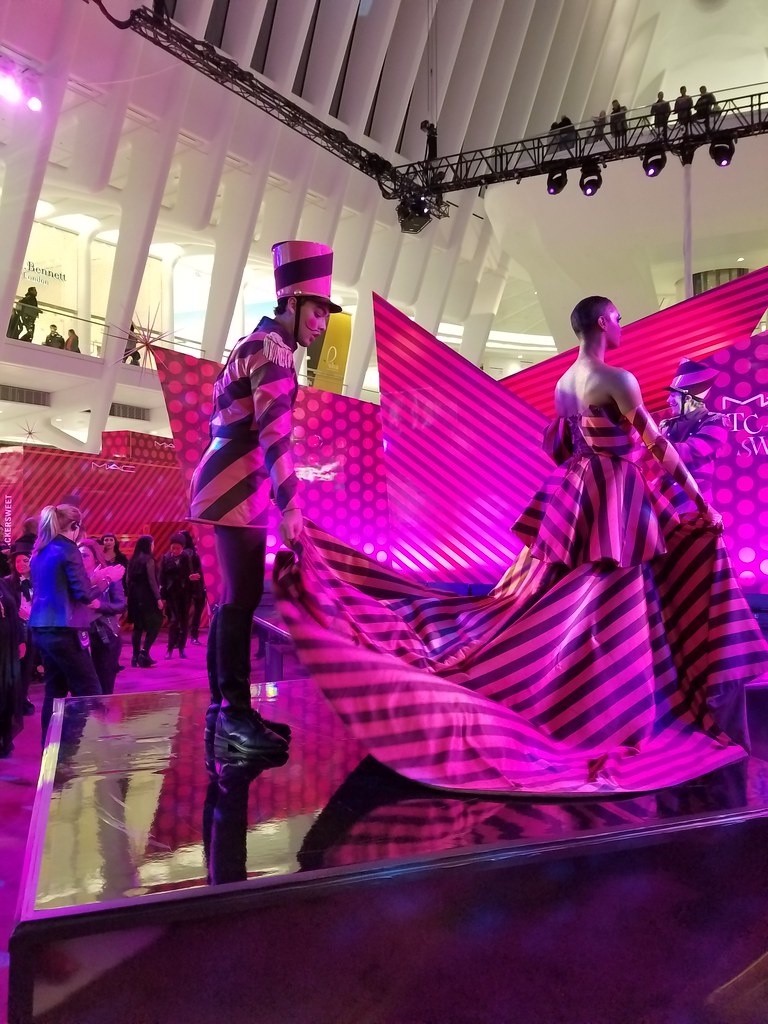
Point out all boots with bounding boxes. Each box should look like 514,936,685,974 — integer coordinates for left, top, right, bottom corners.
204,740,290,884
203,602,292,757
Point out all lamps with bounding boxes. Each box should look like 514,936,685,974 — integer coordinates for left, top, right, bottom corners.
642,142,667,176
579,164,602,196
547,168,568,195
709,133,735,167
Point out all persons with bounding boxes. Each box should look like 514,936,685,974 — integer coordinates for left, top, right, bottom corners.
122,322,140,366
102,534,126,598
673,86,693,119
0,518,44,758
77,539,126,695
185,240,342,755
126,536,164,668
658,357,732,508
65,329,81,353
610,100,628,138
273,295,768,797
650,91,671,128
158,530,207,659
694,86,716,114
592,111,607,142
545,114,577,155
43,325,65,349
20,286,43,343
6,308,22,340
28,504,126,752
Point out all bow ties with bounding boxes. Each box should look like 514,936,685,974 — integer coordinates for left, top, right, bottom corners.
20,579,33,602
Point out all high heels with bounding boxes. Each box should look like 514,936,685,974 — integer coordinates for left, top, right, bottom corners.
132,652,157,667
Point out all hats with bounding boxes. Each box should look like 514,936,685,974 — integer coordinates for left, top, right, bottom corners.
663,357,721,400
9,542,32,555
271,240,342,314
169,533,186,546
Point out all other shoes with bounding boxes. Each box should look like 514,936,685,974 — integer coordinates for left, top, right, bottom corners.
164,647,173,660
190,637,202,646
178,647,188,658
